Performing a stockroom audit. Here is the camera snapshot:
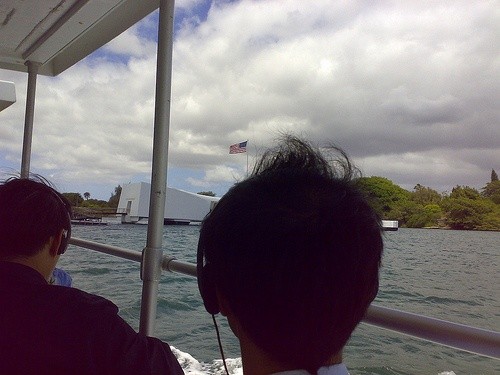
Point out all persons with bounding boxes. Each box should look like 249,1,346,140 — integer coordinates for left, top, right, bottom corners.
0,177,186,375
196,135,384,375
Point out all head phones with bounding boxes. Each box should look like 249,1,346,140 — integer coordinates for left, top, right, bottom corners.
38,182,72,254
196,187,231,314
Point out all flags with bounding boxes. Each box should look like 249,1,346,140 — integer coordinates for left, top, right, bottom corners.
229,140,248,155
